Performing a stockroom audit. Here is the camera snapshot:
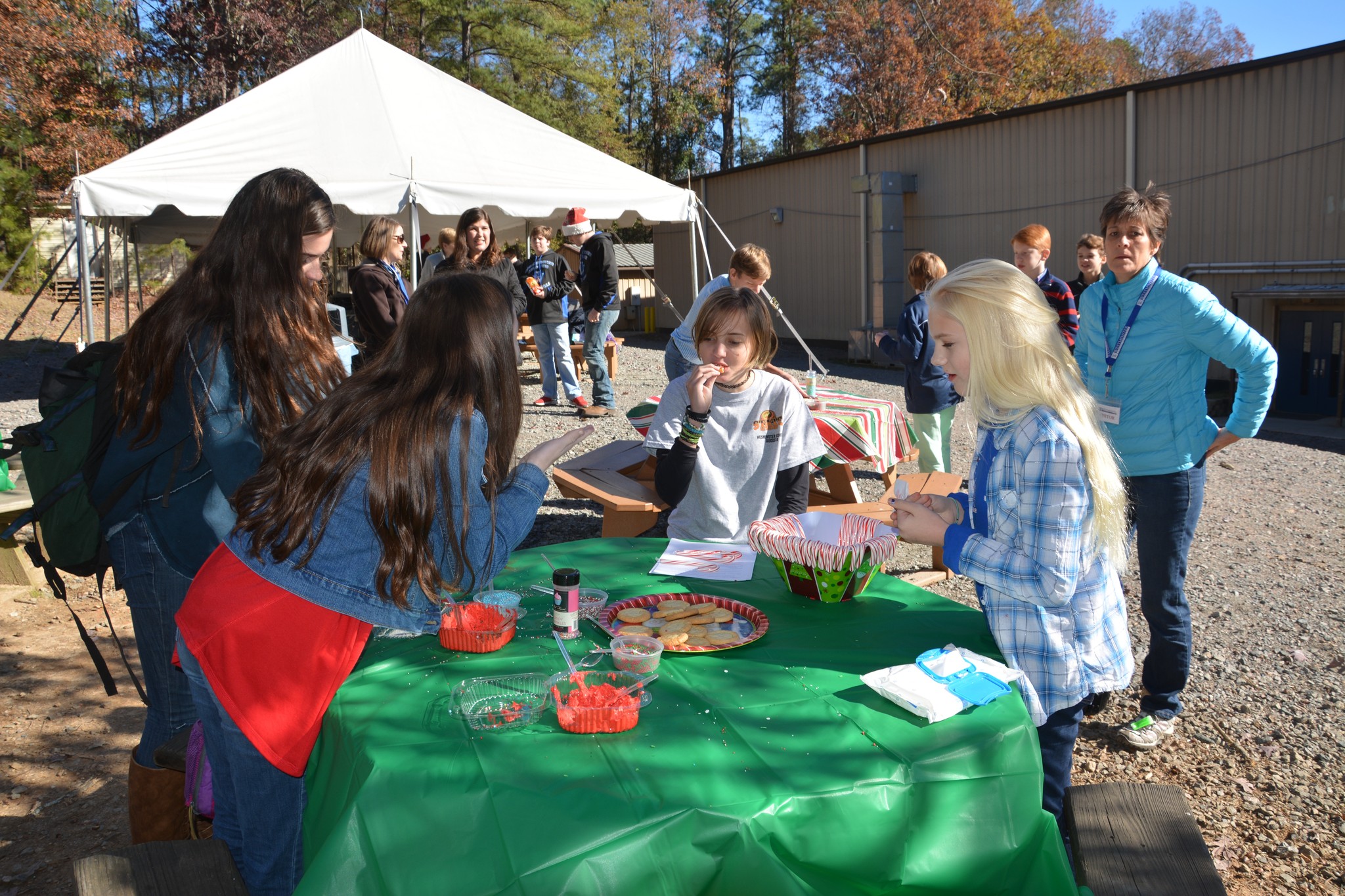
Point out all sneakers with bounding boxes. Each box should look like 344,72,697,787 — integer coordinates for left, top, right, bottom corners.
569,396,589,408
1116,709,1175,750
534,396,560,406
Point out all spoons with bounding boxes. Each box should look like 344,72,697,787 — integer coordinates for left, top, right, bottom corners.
550,652,603,682
590,648,648,655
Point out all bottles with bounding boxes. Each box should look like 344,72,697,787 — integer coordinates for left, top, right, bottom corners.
806,370,816,399
553,568,579,640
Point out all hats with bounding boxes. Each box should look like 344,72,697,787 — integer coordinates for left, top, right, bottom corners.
562,207,592,236
420,234,432,254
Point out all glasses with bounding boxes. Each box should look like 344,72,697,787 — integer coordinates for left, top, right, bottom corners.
392,234,404,244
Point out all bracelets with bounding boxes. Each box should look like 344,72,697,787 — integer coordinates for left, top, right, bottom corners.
679,404,711,444
948,497,959,524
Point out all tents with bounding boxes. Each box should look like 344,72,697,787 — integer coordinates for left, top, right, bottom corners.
65,26,706,392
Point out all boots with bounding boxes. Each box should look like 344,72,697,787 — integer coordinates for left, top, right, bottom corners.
128,743,213,845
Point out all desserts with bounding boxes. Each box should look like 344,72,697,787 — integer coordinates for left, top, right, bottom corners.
616,599,740,647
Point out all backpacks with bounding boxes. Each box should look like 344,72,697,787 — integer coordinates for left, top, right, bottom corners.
12,308,231,578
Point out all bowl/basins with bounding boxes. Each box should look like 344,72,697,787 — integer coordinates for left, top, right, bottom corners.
609,635,664,671
756,511,901,603
448,671,652,735
472,590,521,619
805,399,827,411
371,601,517,653
579,588,608,619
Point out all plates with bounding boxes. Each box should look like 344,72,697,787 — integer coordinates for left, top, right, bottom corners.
598,593,769,652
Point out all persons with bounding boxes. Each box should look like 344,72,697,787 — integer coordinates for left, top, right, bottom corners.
518,225,590,411
875,250,966,474
561,208,623,418
91,165,347,844
886,258,1139,891
171,274,595,896
643,287,829,541
1069,180,1279,750
1011,222,1079,350
1064,232,1106,322
432,206,528,368
346,214,410,358
413,228,529,316
663,242,808,399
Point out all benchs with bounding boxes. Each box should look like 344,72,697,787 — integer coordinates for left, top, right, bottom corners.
524,336,624,384
780,471,962,588
550,440,670,538
855,446,921,493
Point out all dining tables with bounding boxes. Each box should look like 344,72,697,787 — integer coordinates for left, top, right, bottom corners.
293,535,1086,896
627,383,909,504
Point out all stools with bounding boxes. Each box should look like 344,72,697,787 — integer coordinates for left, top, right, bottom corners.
1064,779,1225,894
70,835,247,894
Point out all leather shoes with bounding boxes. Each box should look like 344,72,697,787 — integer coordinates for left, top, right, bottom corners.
577,405,595,415
582,405,616,415
